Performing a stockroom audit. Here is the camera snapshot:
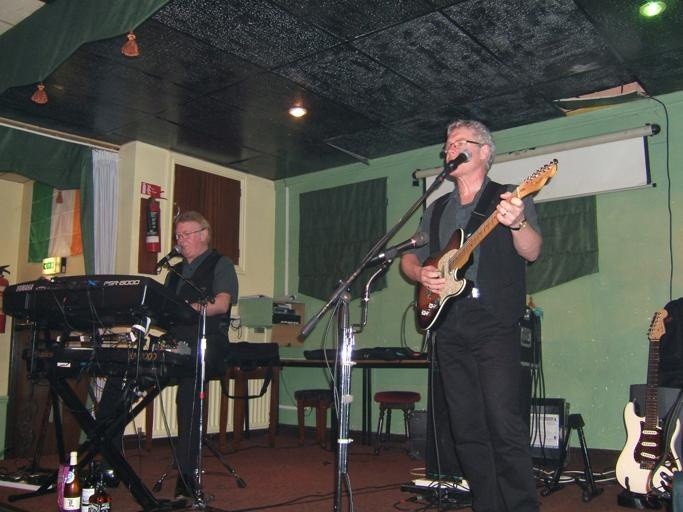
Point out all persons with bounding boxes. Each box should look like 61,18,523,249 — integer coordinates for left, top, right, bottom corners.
97,210,240,503
400,119,545,511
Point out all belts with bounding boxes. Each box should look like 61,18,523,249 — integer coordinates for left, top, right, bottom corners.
458,288,480,300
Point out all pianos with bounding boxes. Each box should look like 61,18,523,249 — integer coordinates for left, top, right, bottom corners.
2,275,203,334
22,340,203,385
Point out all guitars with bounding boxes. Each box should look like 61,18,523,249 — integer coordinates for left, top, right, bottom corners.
616,310,682,494
413,159,559,331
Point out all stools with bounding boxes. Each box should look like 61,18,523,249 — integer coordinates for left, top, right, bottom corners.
151,362,246,494
295,388,334,448
374,391,420,460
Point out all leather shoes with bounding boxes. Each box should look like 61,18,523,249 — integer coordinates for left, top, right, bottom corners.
98,468,120,488
176,480,198,505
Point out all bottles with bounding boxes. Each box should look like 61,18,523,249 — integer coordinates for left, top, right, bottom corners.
63,450,80,512
88,485,111,512
82,477,95,506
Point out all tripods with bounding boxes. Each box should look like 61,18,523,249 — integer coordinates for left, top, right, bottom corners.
153,261,246,502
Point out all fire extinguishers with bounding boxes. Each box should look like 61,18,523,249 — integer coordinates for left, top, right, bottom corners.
144,191,167,253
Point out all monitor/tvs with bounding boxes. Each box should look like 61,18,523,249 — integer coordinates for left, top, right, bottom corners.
528,397,565,466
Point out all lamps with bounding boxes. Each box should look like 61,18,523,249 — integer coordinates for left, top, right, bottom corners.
636,0,667,19
121,32,140,58
288,95,308,118
30,84,48,103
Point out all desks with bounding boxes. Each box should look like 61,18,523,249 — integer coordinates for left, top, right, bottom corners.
278,355,431,446
201,365,280,453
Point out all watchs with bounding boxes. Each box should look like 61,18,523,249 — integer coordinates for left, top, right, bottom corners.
510,219,528,233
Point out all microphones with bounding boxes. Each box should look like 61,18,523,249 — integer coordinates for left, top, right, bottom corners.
437,148,472,186
370,232,429,269
154,244,181,272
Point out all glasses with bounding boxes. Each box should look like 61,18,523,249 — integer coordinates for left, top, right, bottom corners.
176,227,208,239
445,139,481,152
